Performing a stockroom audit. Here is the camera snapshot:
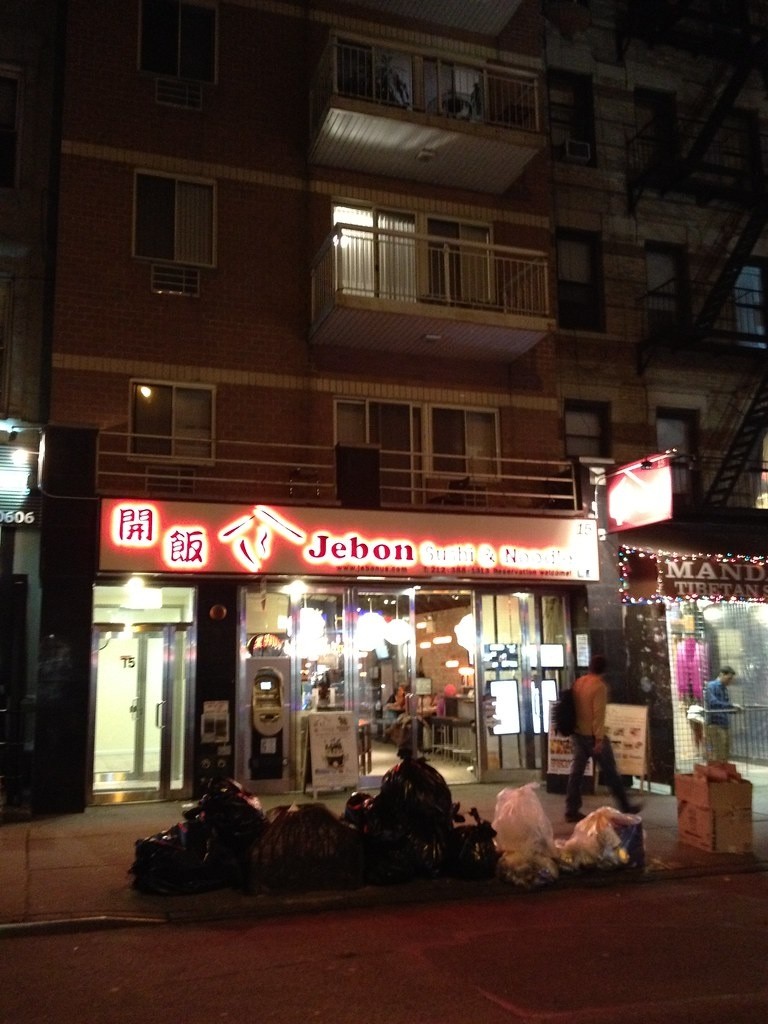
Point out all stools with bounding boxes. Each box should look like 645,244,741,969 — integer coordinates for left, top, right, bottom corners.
429,714,475,766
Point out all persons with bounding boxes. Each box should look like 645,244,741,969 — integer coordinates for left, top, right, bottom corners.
318,681,330,706
563,654,644,823
704,666,743,763
388,685,405,703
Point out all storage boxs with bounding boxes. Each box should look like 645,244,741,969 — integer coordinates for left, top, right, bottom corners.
674,773,753,854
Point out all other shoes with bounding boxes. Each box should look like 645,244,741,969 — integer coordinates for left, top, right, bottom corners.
620,804,641,815
563,808,586,822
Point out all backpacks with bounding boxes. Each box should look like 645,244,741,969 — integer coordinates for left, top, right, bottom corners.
552,683,577,736
